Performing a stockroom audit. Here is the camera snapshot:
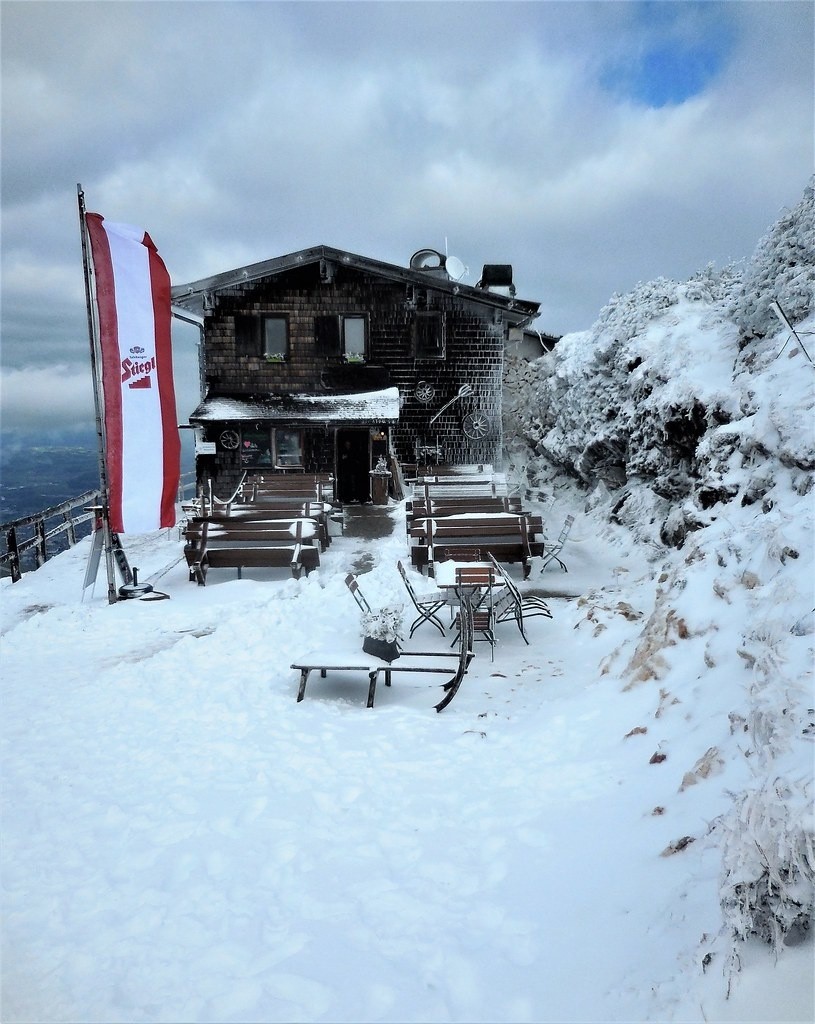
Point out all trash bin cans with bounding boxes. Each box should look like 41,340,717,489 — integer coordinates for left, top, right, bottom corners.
369,469,393,505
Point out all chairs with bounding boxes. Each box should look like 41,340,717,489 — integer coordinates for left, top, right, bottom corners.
540,514,575,574
341,547,554,663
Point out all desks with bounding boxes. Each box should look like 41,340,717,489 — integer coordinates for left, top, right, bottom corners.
436,570,507,648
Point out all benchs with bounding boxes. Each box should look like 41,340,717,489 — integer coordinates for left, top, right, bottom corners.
177,467,544,579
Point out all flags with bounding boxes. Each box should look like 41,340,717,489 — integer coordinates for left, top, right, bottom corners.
86,212,182,534
